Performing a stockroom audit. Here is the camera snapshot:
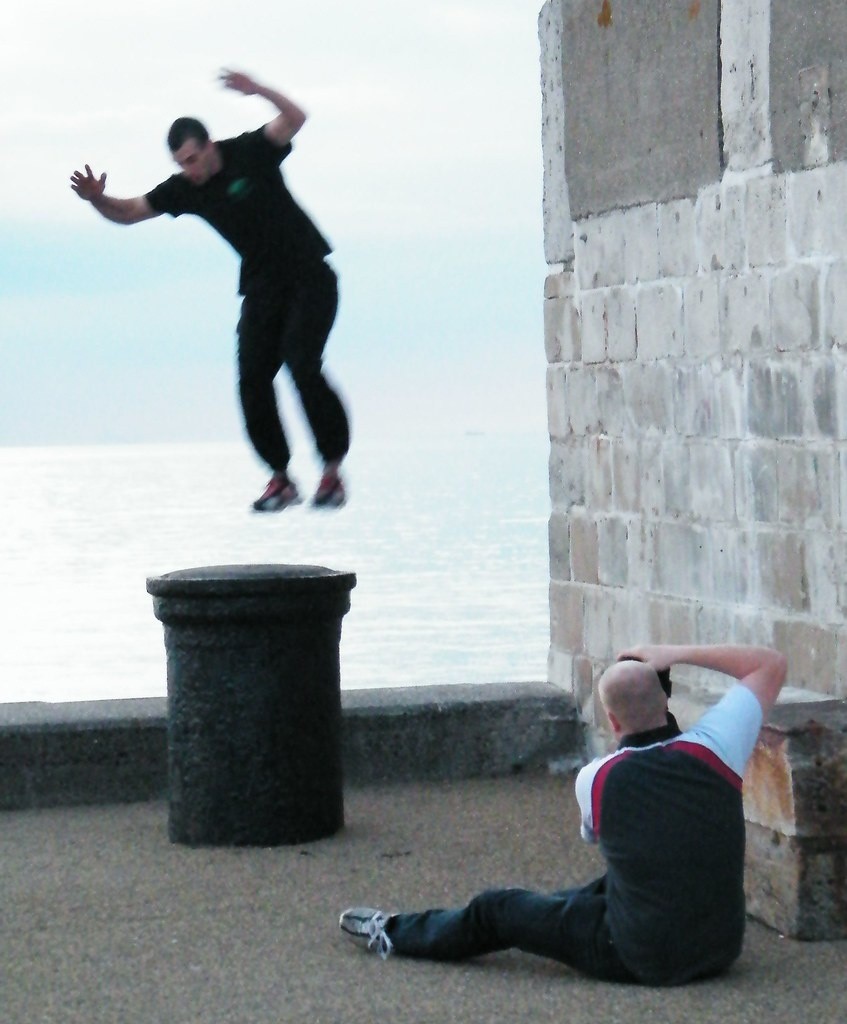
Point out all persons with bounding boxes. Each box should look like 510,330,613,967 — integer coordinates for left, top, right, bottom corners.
71,69,352,511
338,646,787,987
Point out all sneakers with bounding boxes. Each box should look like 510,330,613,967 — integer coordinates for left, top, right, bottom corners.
339,907,400,961
254,473,298,511
313,475,345,506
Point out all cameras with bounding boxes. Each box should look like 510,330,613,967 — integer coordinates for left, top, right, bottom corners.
624,657,672,698
